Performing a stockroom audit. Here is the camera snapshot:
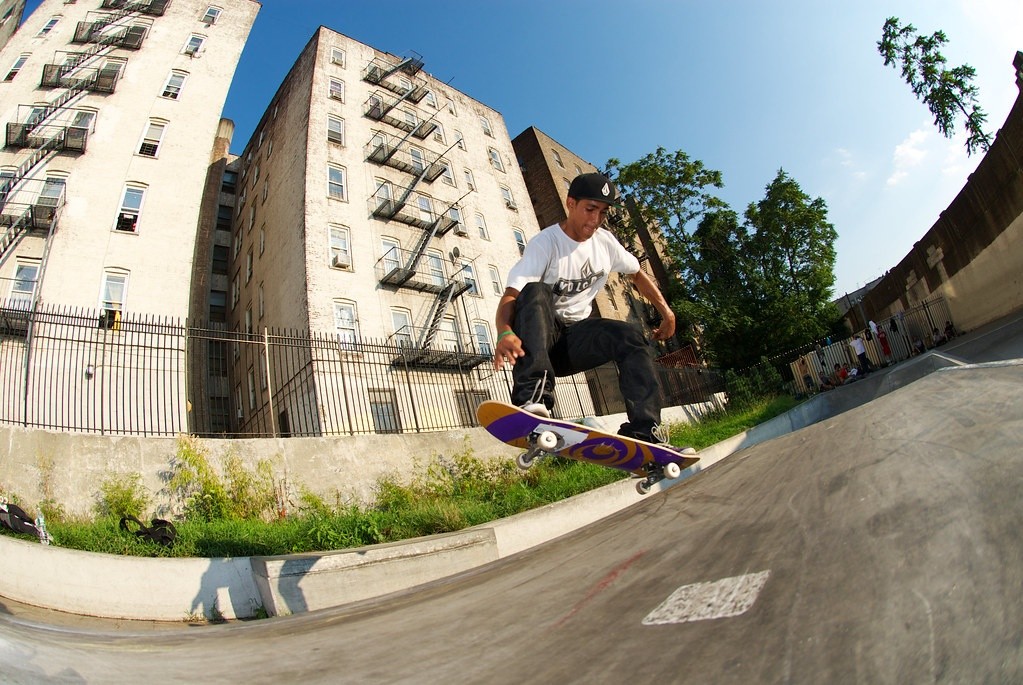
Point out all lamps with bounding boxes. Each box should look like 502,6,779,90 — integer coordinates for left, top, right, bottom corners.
86,365,94,376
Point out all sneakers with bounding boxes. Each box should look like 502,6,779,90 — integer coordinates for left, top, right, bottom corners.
651,421,695,455
520,370,551,419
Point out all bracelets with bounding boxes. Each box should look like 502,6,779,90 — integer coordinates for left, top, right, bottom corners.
497,332,514,344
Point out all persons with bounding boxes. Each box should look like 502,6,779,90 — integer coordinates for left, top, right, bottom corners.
494,172,696,455
819,363,864,391
932,328,947,346
944,321,955,341
926,333,935,350
799,355,814,392
912,336,926,354
846,333,868,373
878,332,897,364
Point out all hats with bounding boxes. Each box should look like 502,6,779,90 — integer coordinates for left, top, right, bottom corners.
568,173,626,209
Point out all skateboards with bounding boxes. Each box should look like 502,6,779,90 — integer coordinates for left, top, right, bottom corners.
474,399,701,496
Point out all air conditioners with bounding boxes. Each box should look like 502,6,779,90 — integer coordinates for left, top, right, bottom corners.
185,45,198,55
332,57,343,65
333,92,341,100
333,254,352,268
508,200,518,210
433,132,443,142
454,224,467,236
